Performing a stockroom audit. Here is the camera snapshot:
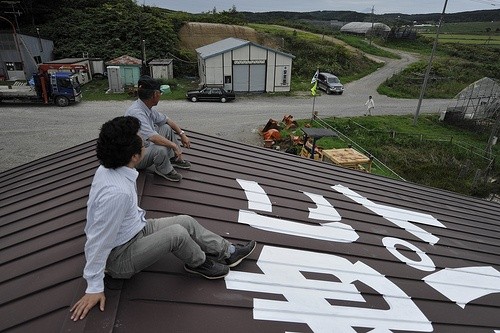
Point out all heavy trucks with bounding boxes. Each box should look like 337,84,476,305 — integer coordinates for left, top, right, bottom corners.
0,67,83,106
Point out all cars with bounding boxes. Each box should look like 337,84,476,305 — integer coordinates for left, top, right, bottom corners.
185,83,236,103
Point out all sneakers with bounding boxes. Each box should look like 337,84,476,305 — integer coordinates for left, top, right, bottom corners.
170,156,192,168
155,167,182,181
225,240,256,267
183,258,230,279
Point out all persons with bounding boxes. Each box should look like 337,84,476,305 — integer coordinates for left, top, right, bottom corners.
363,95,375,116
69,115,257,321
124,75,191,181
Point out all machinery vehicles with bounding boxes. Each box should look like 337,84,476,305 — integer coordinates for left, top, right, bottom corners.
301,127,338,162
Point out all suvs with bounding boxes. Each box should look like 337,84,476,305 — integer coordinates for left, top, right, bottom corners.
316,72,344,95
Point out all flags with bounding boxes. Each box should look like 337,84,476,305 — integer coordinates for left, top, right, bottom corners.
310,71,319,95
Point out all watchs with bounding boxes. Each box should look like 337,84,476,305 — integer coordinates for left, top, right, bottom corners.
180,131,185,136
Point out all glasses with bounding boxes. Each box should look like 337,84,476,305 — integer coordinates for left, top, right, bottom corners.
160,91,163,95
